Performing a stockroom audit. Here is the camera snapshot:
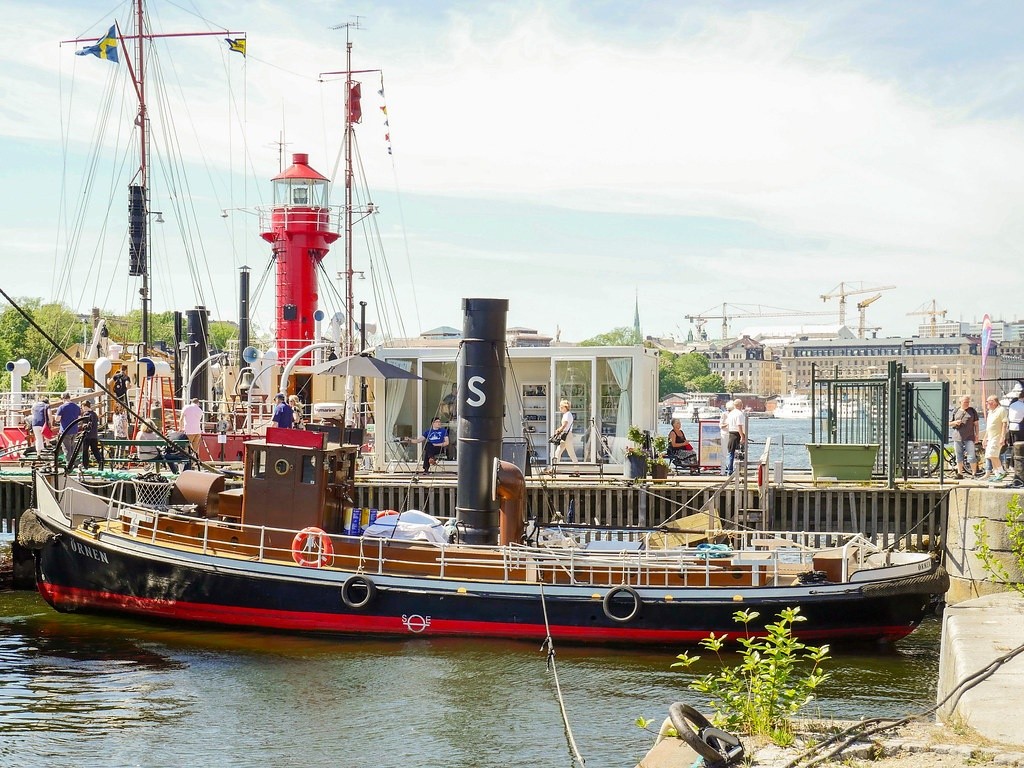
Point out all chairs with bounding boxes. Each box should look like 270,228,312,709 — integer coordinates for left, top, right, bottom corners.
429,449,447,472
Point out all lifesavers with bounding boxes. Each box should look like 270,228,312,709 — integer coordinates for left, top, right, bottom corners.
377,510,398,518
603,584,642,623
341,575,377,609
291,527,334,570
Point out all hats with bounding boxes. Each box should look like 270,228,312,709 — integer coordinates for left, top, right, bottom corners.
61,393,70,398
42,397,48,403
274,394,283,401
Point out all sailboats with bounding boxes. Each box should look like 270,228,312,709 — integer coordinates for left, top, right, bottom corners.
0,0,951,656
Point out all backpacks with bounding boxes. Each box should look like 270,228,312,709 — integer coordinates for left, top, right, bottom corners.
113,374,126,397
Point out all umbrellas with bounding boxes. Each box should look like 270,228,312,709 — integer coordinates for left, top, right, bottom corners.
292,351,423,380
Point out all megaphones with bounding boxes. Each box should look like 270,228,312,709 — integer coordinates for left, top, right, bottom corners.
242,346,264,364
6,359,30,376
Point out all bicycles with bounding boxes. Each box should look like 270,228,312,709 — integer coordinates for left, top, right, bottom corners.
906,436,987,476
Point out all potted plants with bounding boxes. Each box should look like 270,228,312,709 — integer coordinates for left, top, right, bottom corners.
620,424,650,484
649,435,673,484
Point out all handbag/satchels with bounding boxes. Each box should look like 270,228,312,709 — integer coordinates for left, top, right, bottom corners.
41,423,53,439
554,433,567,445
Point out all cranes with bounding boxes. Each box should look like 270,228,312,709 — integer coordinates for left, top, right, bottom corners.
852,293,881,340
685,301,842,344
905,299,947,338
819,281,897,326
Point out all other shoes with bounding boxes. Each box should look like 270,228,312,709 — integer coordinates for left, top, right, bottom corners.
690,471,700,475
423,470,428,475
429,458,434,465
954,470,1008,482
545,470,555,476
569,473,580,477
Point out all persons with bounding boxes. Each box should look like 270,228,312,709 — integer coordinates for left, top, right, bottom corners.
113,403,128,470
270,393,304,429
107,365,131,423
546,401,580,477
1008,391,1024,441
31,396,53,459
719,399,745,476
949,396,980,479
404,418,450,475
161,430,190,474
135,418,161,474
441,383,457,419
180,398,202,451
55,393,81,468
667,418,700,475
82,400,104,470
982,395,1009,483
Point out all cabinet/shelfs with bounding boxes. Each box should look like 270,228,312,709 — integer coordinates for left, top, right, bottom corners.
521,380,549,464
599,382,622,456
559,382,587,461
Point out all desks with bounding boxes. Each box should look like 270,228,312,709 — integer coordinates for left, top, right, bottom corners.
384,440,411,473
812,547,860,582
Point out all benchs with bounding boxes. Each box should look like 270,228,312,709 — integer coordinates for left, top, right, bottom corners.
99,439,198,473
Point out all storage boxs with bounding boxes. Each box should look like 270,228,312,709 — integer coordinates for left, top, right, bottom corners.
358,507,377,535
342,507,362,536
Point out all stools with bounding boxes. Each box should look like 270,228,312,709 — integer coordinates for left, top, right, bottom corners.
907,440,931,478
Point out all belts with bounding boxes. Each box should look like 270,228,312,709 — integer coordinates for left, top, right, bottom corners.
170,451,180,455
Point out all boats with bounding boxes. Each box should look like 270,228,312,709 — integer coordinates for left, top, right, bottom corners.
827,392,868,419
671,389,723,420
773,388,829,420
